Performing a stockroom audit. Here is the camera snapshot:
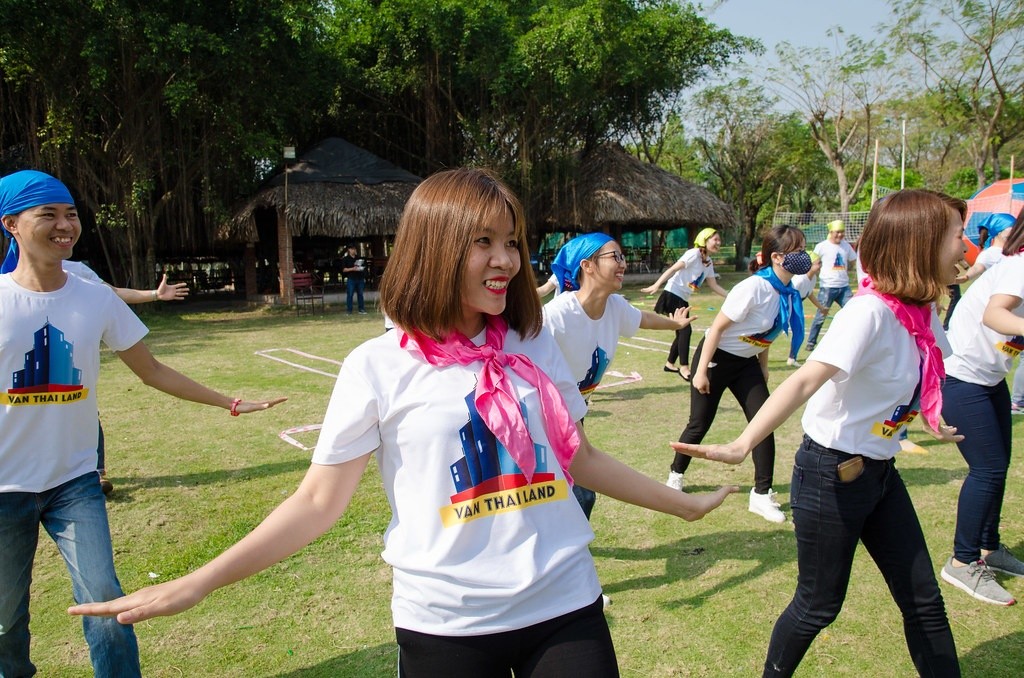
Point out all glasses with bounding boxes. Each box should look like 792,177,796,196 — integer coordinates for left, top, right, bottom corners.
595,251,625,263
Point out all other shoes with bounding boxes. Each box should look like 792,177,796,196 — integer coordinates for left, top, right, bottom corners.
787,359,801,367
664,365,679,372
1011,402,1024,415
679,370,691,381
805,345,814,351
99,475,113,495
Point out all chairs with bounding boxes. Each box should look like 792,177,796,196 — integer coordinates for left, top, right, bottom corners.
621,246,665,274
364,256,389,286
291,273,325,317
164,268,232,291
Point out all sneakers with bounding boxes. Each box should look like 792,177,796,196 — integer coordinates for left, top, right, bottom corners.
666,471,683,491
941,556,1015,605
749,487,786,522
980,543,1024,575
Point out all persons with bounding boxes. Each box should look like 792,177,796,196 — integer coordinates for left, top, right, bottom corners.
666,225,811,524
806,218,857,352
669,189,969,678
898,202,1024,606
786,247,830,369
64,257,190,492
342,243,367,314
69,165,739,678
383,231,700,608
0,169,287,678
639,226,730,382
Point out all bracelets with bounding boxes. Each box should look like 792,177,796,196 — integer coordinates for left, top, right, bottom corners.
153,290,157,302
231,399,241,417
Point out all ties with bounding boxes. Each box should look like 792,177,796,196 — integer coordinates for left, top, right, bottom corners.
852,275,946,435
401,310,582,488
753,267,805,356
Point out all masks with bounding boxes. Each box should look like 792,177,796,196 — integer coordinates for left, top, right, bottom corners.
778,252,812,275
